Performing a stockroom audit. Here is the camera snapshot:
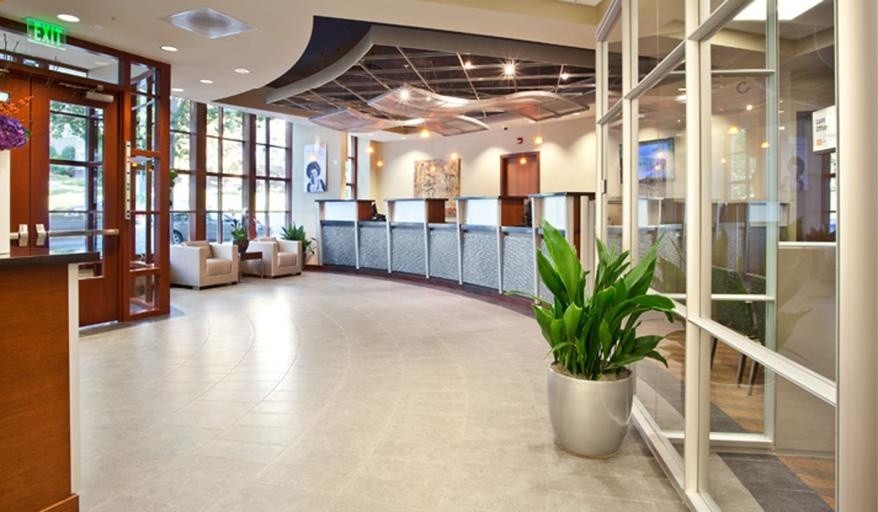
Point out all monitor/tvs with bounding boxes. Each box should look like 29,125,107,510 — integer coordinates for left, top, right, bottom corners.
619,137,676,184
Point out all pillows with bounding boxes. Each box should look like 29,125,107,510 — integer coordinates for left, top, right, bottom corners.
255,236,280,251
182,240,212,257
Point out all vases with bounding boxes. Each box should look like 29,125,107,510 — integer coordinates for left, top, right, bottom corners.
0,149,11,254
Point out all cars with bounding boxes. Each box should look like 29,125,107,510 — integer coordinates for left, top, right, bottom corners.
167,208,266,243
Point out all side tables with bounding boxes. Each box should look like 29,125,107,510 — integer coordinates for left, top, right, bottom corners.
239,251,263,281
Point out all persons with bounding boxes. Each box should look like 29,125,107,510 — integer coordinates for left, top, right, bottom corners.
306,161,326,192
783,156,804,190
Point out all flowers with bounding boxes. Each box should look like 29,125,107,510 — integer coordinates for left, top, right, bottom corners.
0,32,59,150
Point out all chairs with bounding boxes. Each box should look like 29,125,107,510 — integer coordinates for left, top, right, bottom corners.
241,239,303,281
169,241,238,290
708,267,766,394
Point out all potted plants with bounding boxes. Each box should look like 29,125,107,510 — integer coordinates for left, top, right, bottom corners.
230,213,249,252
503,219,675,459
279,221,316,270
